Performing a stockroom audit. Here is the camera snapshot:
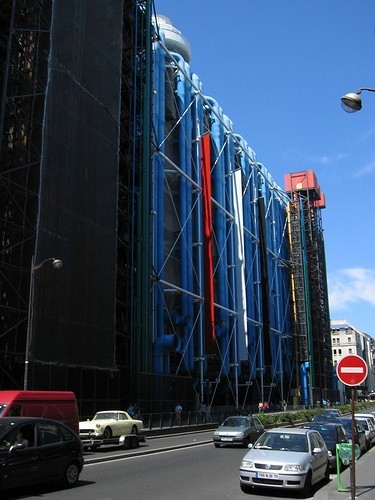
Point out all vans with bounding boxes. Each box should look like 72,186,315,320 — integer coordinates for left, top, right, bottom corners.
0,390,80,450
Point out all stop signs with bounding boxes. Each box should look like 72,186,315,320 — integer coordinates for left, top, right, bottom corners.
336,354,368,387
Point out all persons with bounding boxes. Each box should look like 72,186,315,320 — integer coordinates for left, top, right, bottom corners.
283,400,288,412
132,402,141,420
4,430,29,452
174,402,182,425
262,401,269,413
127,401,134,418
258,400,264,413
200,401,207,422
206,401,213,423
327,400,331,408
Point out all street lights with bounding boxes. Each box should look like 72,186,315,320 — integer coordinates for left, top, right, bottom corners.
340,86,375,113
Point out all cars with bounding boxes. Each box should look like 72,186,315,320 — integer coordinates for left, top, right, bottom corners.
311,412,341,423
321,417,366,453
301,422,348,472
0,417,84,498
239,427,330,494
352,417,375,448
78,410,143,439
212,416,264,448
354,414,375,421
323,409,341,416
